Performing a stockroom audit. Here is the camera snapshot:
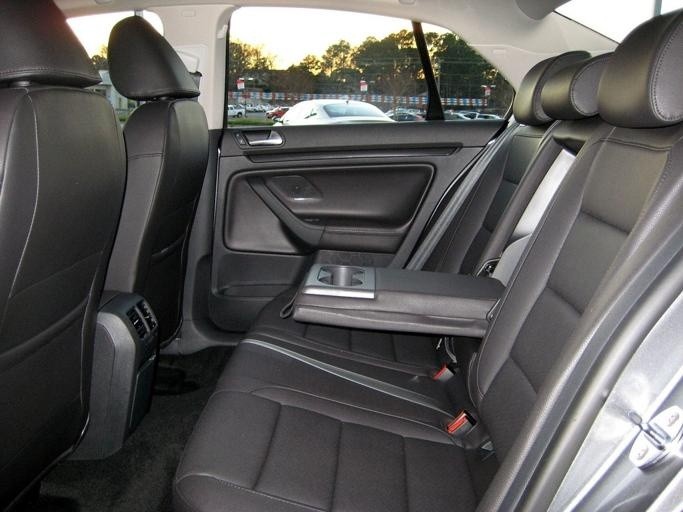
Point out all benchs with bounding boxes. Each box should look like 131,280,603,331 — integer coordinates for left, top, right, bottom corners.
171,9,683,511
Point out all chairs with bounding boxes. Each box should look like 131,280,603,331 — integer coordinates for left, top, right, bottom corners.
1,1,129,512
104,16,208,351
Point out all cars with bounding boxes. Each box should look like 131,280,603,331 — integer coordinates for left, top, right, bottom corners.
265,106,290,119
271,98,397,126
386,106,504,122
227,103,273,119
